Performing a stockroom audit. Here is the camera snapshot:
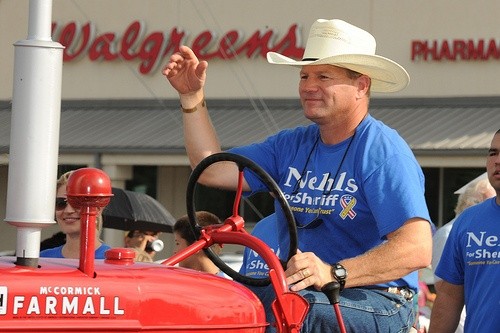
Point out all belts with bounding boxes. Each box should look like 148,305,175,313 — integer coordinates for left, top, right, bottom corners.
376,287,410,299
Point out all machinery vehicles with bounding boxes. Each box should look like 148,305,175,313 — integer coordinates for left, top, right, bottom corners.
0,1,347,333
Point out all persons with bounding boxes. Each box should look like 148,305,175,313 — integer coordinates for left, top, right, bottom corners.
40,169,112,260
162,19,436,333
237,211,284,278
173,211,233,279
123,230,161,262
409,128,500,333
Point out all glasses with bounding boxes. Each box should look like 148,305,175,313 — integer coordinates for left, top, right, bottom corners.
56,197,67,211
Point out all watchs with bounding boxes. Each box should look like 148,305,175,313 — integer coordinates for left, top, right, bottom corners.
330,263,347,292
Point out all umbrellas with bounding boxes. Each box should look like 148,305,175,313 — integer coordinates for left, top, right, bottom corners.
100,187,177,234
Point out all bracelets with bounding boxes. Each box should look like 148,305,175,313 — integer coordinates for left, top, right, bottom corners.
178,94,207,113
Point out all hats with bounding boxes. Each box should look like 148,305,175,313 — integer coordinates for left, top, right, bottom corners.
267,18,411,93
